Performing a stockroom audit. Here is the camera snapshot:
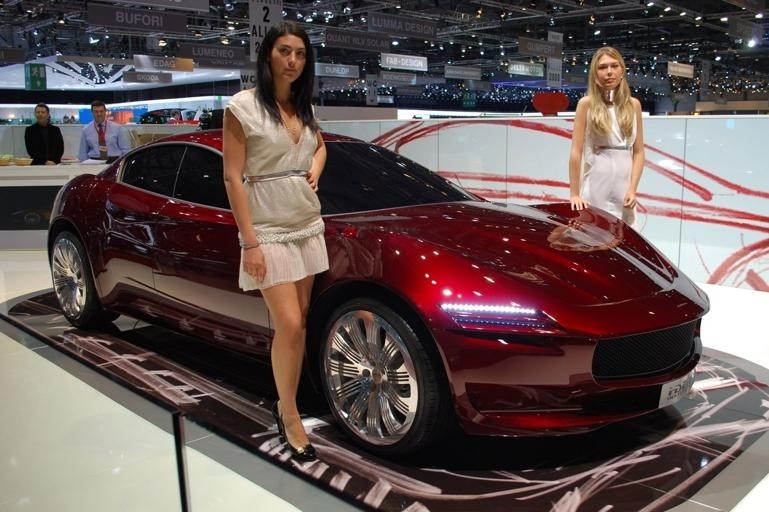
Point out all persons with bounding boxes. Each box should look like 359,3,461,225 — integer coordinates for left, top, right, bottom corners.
79,100,130,164
547,209,626,252
167,110,181,123
322,228,383,281
25,103,65,165
568,46,645,231
223,21,329,462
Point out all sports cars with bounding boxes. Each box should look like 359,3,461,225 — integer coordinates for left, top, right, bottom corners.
46,127,711,461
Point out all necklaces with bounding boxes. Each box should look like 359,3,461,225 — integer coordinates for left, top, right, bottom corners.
280,109,297,135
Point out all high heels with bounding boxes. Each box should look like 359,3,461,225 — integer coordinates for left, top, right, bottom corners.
272,401,316,461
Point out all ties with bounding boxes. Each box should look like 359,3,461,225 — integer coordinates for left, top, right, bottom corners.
97,123,107,160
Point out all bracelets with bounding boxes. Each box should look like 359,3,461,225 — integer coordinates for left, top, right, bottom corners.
240,242,259,250
